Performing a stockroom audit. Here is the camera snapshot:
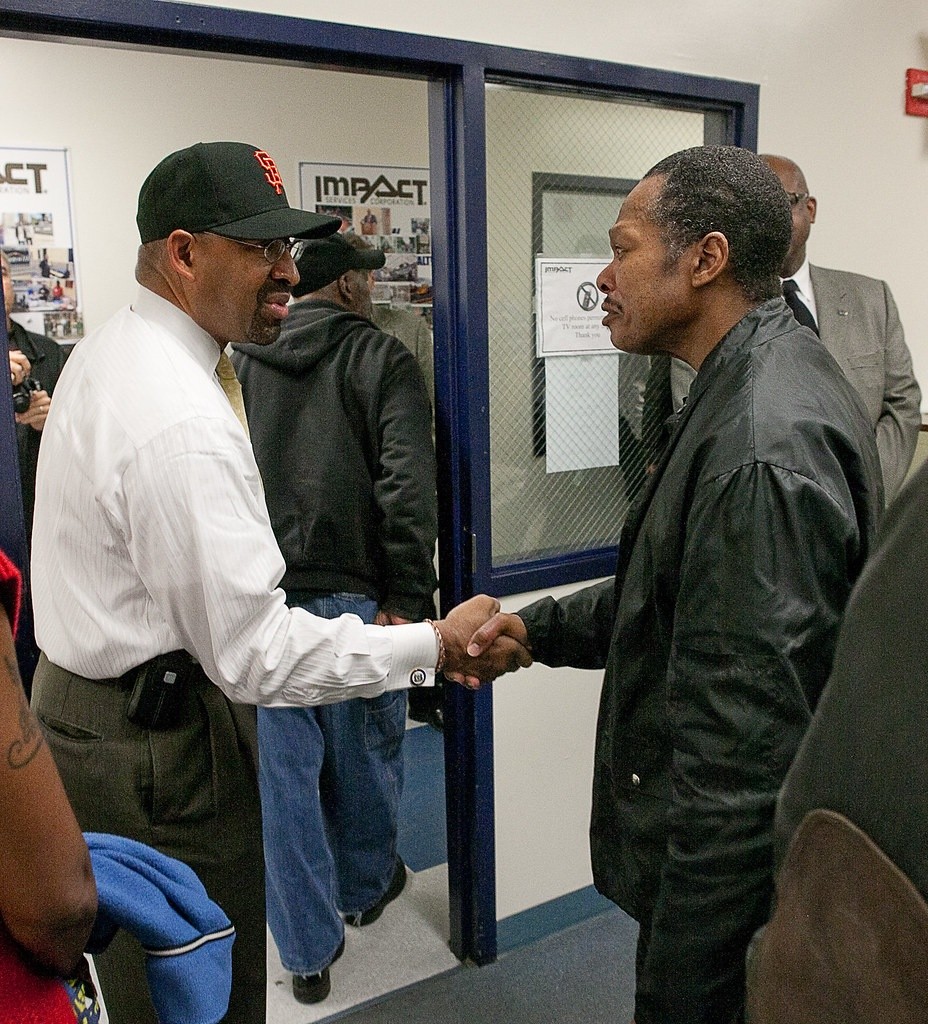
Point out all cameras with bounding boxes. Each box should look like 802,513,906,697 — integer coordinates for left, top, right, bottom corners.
11,376,42,414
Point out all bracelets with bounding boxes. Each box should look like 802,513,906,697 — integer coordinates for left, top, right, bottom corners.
424,617,445,675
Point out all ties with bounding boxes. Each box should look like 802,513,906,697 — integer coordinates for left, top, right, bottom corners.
782,280,820,340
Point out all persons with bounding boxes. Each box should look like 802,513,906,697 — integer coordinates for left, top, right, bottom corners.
446,145,928,1024
0,141,533,1024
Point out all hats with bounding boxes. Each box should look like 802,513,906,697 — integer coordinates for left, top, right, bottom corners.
288,231,386,298
136,140,343,245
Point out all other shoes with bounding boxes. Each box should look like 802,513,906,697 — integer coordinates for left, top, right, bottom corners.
409,706,444,733
292,932,346,1002
344,853,407,926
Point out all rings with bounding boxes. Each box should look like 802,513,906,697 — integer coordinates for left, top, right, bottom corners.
40,406,44,415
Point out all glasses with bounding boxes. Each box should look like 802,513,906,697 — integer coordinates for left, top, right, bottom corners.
202,230,305,263
786,192,808,208
